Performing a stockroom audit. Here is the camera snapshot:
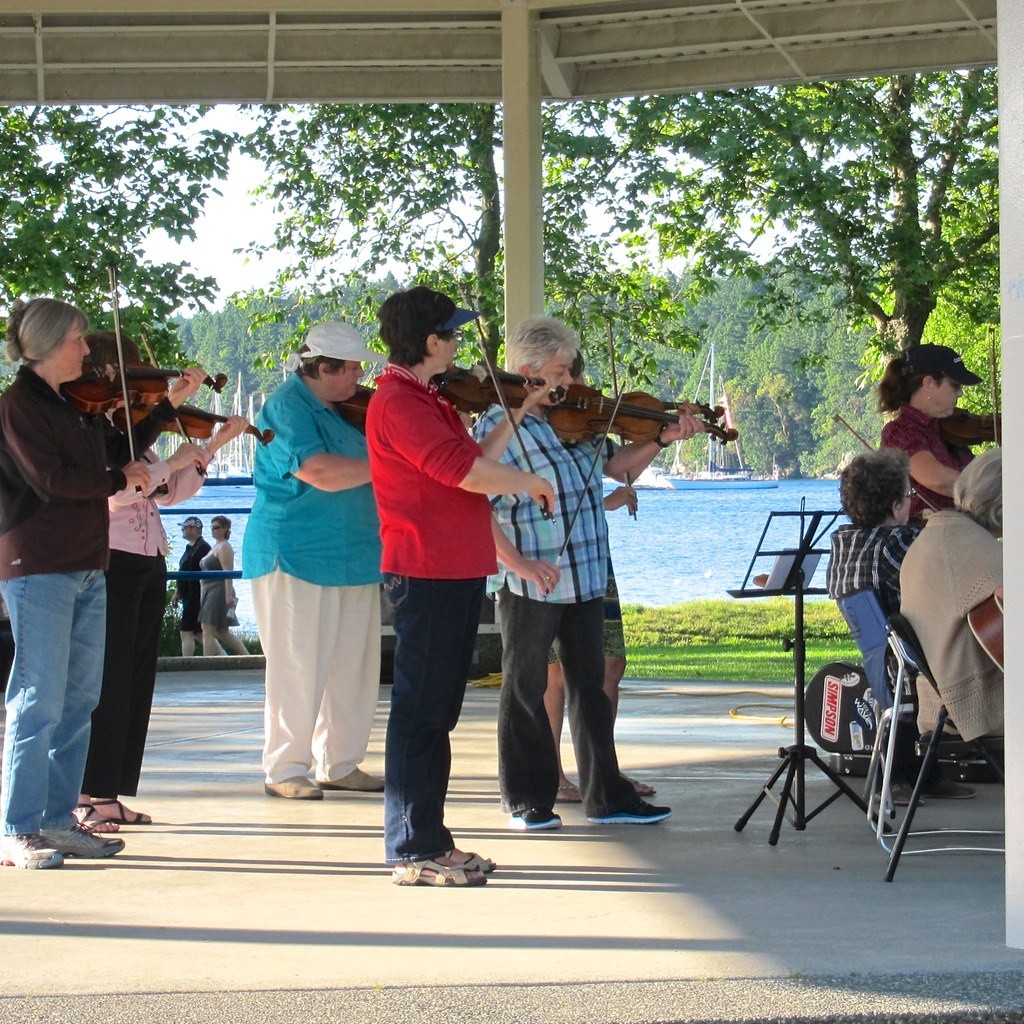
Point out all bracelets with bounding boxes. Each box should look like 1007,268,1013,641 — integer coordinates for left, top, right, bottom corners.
654,435,674,449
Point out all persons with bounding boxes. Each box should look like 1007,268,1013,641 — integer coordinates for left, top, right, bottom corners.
365,284,569,889
477,315,705,832
241,320,388,800
0,296,249,869
826,342,1004,802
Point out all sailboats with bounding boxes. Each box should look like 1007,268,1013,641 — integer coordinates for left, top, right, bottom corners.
149,359,287,497
658,342,779,490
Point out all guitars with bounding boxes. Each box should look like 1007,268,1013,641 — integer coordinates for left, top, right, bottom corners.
967,592,1004,673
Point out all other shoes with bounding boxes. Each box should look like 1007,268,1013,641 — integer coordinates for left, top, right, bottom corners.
913,773,978,797
875,782,925,807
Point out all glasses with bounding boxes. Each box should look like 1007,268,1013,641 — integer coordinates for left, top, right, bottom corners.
210,525,220,530
905,486,916,501
943,380,963,392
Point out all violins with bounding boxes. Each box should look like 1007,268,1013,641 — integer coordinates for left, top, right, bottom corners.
546,383,739,445
616,391,725,424
937,407,1001,447
334,383,478,425
111,402,274,446
60,356,228,414
430,364,566,414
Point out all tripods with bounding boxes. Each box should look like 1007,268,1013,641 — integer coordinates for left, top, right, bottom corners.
722,510,897,847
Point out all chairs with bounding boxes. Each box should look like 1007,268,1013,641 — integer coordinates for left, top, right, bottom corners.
837,585,1004,886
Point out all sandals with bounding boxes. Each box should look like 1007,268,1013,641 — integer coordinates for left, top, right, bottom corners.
71,804,120,833
392,847,497,886
92,799,152,824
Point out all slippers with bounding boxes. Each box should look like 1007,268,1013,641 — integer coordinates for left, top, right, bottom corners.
555,783,583,803
618,771,656,796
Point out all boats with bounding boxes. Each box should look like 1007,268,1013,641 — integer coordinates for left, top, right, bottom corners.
602,463,676,492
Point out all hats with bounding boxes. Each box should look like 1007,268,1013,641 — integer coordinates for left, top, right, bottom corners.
295,319,384,363
177,516,203,530
907,345,984,386
425,290,481,332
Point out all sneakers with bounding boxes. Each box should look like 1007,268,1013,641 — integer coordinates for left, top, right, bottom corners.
509,808,562,830
39,823,125,857
265,777,324,800
587,798,673,824
0,832,63,870
317,767,384,792
